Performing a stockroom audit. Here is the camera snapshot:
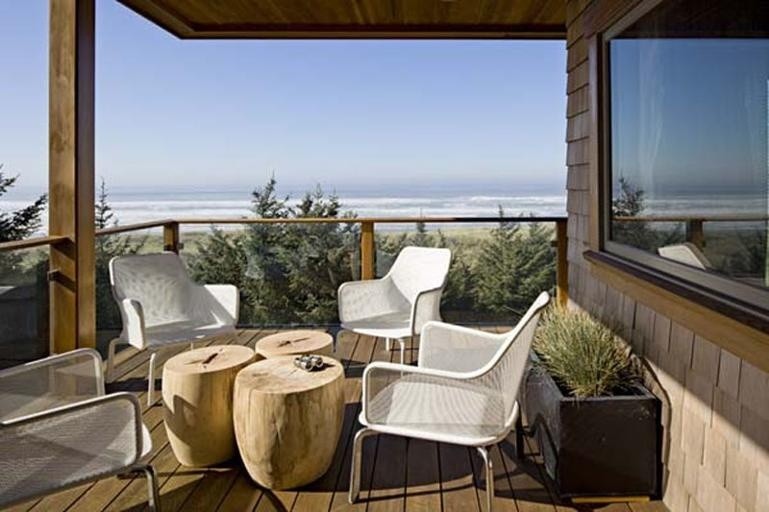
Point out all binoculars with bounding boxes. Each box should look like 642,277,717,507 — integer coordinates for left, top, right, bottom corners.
293,354,323,371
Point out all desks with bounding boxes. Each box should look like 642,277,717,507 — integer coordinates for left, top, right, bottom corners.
233,353,345,491
161,343,253,467
256,329,336,360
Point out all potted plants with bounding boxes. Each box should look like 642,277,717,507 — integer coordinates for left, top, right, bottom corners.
518,301,666,502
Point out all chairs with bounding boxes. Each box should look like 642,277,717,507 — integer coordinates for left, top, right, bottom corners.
1,347,162,512
658,240,711,271
335,246,452,380
347,289,550,511
107,250,240,407
